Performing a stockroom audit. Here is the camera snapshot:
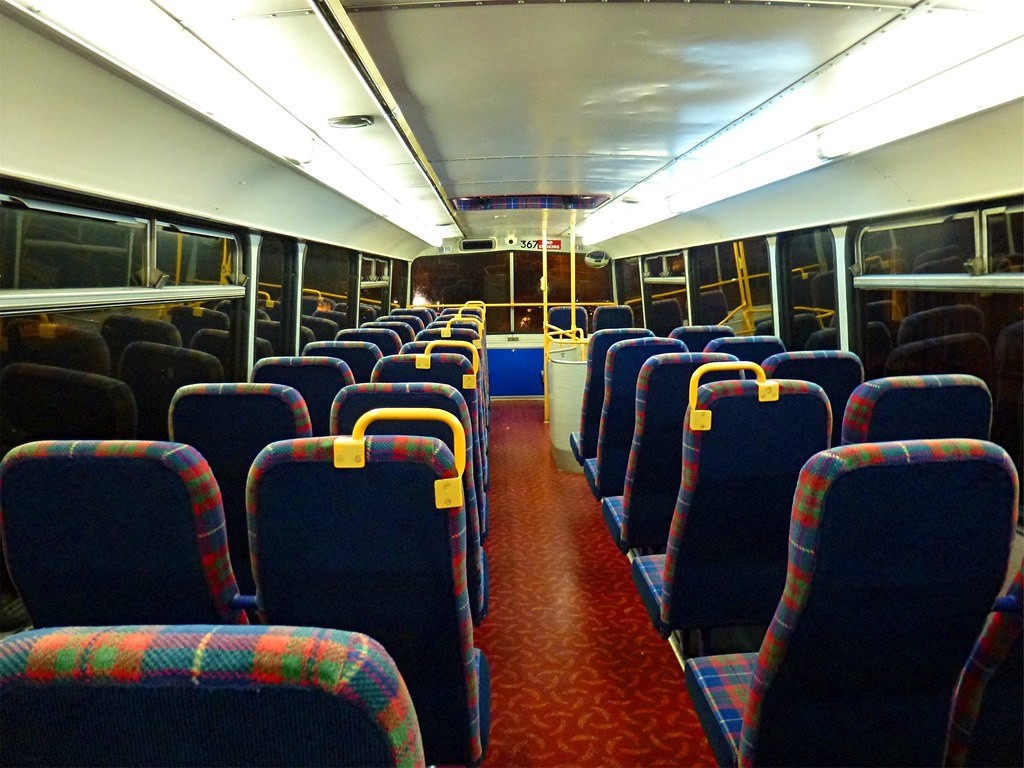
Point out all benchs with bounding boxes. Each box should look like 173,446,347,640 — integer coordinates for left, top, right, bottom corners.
602,351,865,562
629,361,995,669
583,336,786,502
568,325,737,466
0,300,491,768
683,437,1024,768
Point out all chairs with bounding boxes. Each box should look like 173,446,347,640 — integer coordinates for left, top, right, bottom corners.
592,305,635,333
546,306,589,339
